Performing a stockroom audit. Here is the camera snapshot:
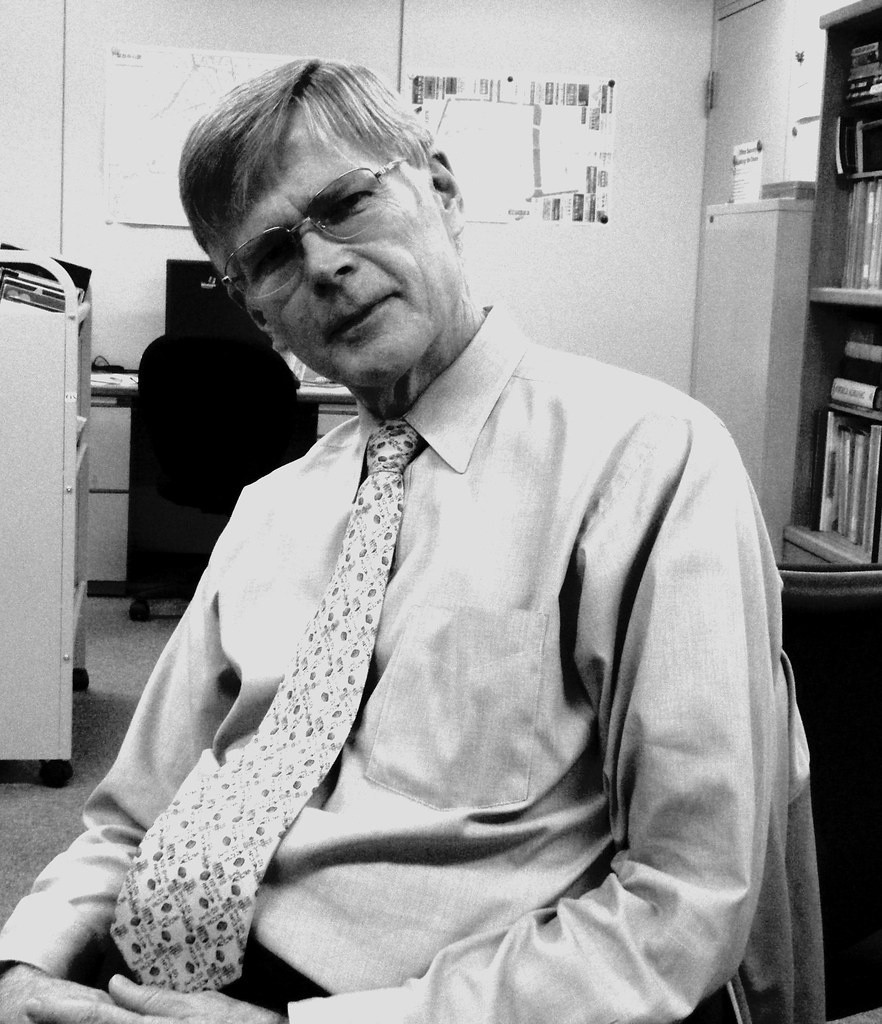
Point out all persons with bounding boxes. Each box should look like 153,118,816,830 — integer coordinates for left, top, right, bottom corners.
0,62,785,1024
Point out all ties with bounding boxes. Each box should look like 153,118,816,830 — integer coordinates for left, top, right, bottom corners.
109,420,417,993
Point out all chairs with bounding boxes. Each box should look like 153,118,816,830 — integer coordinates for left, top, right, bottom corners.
782,570,882,1024
126,332,295,625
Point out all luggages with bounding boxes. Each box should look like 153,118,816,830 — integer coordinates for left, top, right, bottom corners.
126,324,319,622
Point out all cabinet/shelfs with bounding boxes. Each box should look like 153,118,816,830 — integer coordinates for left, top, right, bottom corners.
779,0,882,567
0,247,96,788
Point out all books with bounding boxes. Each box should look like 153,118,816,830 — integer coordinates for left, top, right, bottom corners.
808,319,882,556
839,31,882,291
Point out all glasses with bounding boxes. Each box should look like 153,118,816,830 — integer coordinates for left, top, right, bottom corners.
221,156,407,300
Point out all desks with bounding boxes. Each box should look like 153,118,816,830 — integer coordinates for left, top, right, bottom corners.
91,371,356,405
